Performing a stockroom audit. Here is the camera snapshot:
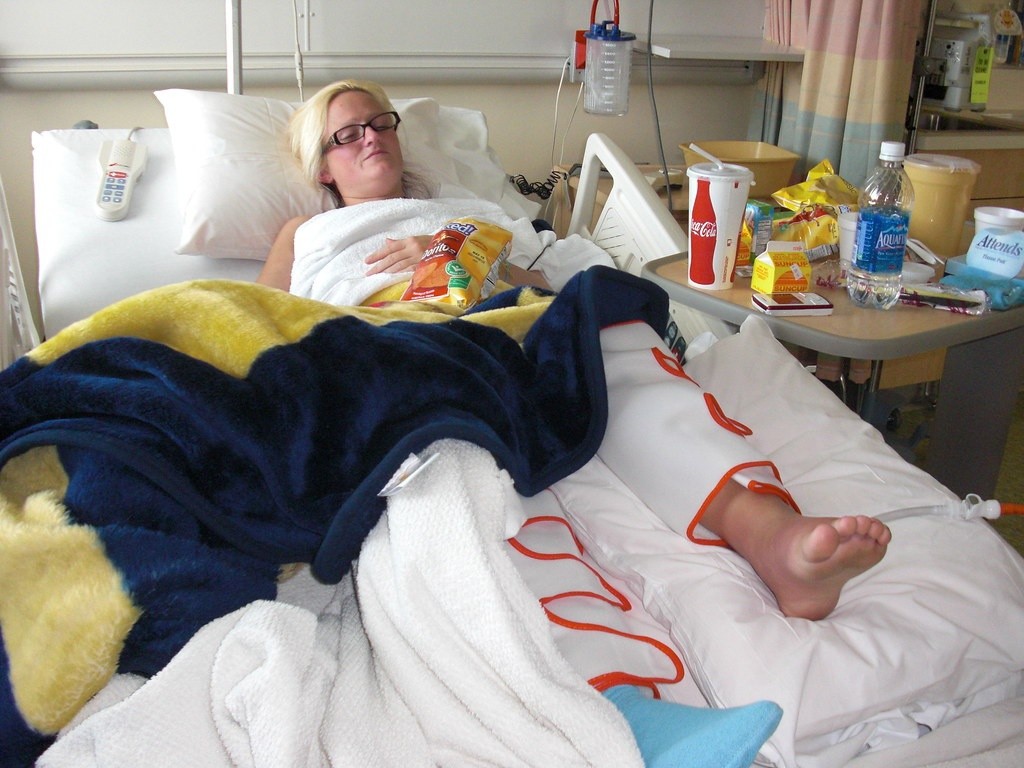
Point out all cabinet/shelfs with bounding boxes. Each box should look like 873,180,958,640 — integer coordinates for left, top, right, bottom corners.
550,164,782,240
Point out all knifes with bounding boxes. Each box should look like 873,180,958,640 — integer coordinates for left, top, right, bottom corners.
846,290,983,309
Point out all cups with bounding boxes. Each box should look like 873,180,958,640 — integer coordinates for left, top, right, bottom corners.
686,163,754,293
838,212,883,277
583,30,637,117
901,153,983,284
974,207,1024,237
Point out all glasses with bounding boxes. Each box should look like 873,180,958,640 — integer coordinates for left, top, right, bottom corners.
322,111,402,152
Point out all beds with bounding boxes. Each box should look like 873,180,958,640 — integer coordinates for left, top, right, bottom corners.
31,107,1024,768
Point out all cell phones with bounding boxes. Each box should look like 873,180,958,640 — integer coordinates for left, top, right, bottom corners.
751,292,834,316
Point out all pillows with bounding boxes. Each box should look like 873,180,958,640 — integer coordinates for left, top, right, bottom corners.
152,87,463,263
547,311,1024,768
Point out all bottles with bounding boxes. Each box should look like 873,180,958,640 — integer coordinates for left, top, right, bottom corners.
848,141,915,312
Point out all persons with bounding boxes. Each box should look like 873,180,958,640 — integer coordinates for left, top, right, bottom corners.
252,79,892,623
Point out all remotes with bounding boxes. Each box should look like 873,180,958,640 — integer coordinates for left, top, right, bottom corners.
95,139,138,222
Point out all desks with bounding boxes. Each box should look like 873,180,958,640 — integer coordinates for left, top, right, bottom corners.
639,220,1024,466
